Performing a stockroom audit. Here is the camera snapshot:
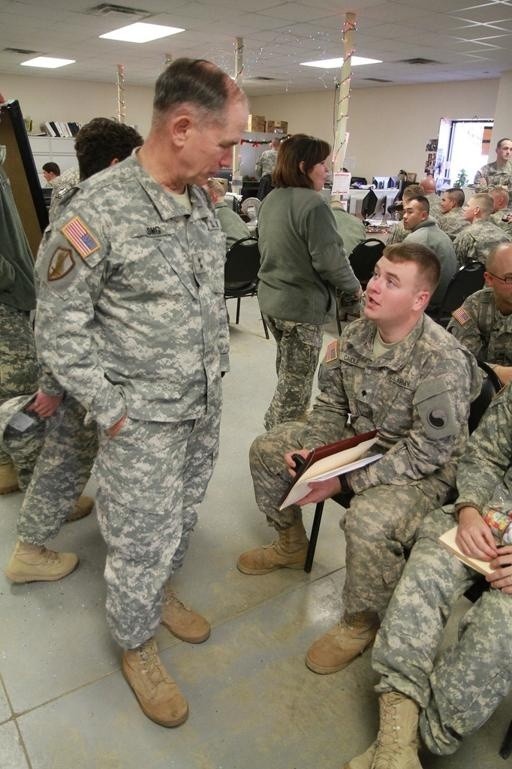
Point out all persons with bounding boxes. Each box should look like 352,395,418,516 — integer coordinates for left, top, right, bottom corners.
0,143,95,529
27,157,65,192
394,138,512,385
236,239,485,677
28,54,256,731
3,114,145,587
257,133,365,430
205,180,254,288
345,377,511,768
330,201,365,320
257,138,280,175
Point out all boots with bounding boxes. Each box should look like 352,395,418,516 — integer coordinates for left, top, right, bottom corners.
66,494,95,524
160,593,212,644
305,609,382,676
5,540,79,585
120,635,190,729
235,520,311,576
342,690,423,769
0,463,19,495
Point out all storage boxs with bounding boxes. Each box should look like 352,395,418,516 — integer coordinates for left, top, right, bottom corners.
246,115,265,132
265,120,289,133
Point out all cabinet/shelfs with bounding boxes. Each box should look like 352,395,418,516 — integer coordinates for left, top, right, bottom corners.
29,135,80,191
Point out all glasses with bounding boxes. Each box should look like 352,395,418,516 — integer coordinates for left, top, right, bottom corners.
488,272,512,284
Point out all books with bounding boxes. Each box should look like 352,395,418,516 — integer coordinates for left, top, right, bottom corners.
435,508,505,588
276,424,385,513
